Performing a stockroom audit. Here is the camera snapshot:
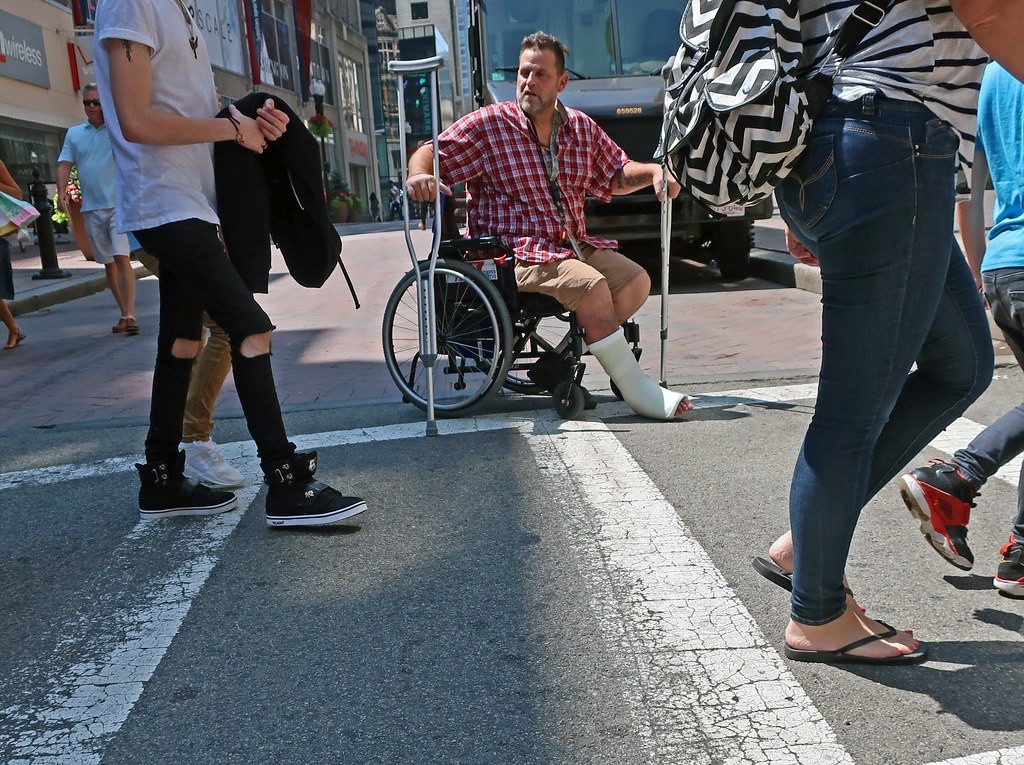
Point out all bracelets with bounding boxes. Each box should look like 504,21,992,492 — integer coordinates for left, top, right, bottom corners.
227,114,246,144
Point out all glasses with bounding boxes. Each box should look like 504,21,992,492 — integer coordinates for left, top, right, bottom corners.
83,98,101,106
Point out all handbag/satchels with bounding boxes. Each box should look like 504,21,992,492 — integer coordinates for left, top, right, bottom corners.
0,191,41,238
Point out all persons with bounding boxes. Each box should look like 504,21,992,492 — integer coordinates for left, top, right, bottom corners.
0,159,26,348
899,59,1024,599
57,0,434,527
751,0,1024,670
406,30,694,420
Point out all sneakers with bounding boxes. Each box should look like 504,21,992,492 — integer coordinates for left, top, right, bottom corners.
134,449,239,518
898,458,974,571
993,535,1024,597
259,450,368,526
177,438,245,487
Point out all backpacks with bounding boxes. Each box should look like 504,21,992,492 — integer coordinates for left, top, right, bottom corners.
653,0,813,219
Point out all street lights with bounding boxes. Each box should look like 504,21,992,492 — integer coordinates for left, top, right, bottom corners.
308,75,332,213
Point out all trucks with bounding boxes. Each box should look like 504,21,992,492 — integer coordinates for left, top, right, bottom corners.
465,1,774,281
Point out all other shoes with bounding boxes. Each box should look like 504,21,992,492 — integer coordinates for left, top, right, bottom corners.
3,327,26,349
112,317,125,333
124,316,140,332
528,353,597,410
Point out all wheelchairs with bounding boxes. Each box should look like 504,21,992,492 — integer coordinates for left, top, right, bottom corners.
379,173,643,420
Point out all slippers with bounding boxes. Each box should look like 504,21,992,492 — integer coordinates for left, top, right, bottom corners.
750,556,854,600
786,619,929,666
